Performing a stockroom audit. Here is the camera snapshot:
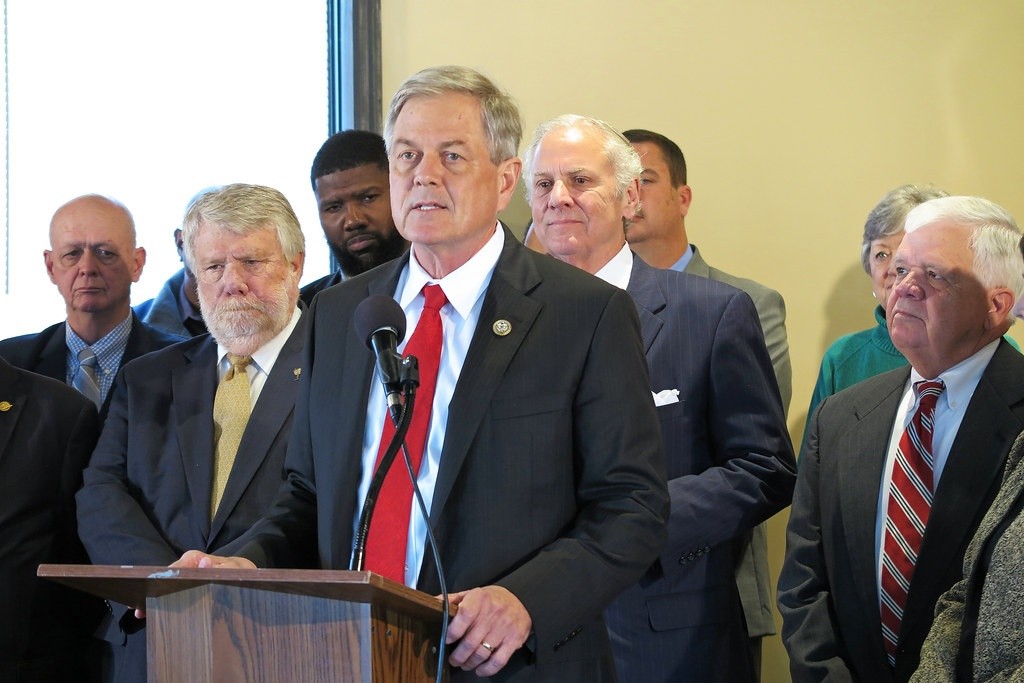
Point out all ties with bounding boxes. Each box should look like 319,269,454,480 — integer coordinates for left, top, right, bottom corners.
210,353,253,522
880,381,946,668
360,284,449,587
72,348,102,415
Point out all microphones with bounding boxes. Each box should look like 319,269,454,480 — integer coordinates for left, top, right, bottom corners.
354,294,407,426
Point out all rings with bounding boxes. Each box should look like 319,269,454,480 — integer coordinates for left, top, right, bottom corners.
481,641,496,652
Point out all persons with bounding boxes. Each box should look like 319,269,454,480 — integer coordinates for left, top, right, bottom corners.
130,66,672,683
75,185,313,683
0,195,191,683
521,116,1023,683
298,130,411,308
